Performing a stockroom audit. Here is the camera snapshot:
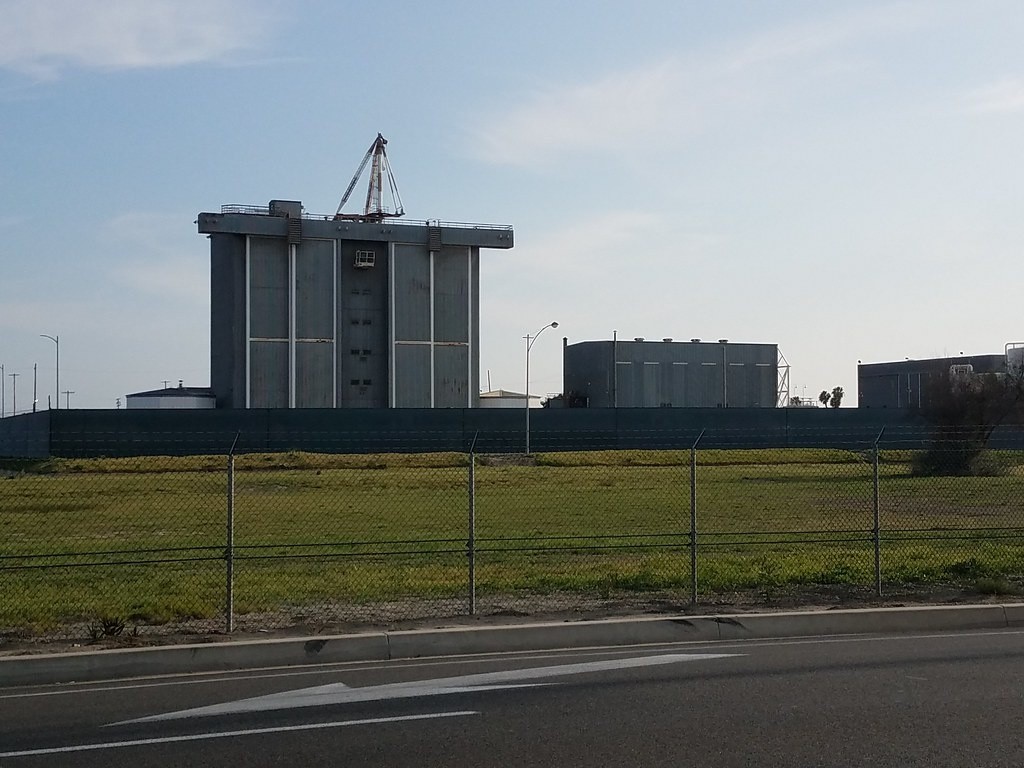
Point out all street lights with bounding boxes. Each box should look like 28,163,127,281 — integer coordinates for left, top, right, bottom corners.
522,321,559,456
2,334,75,419
